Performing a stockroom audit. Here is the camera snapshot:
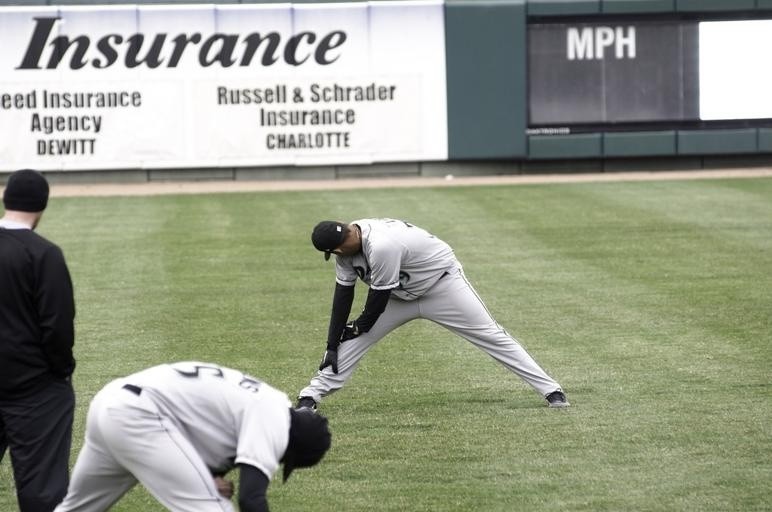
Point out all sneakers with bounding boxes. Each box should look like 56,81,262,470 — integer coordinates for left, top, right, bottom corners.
294,396,317,415
545,387,570,408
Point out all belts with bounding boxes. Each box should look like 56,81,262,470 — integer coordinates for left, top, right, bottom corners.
123,384,142,394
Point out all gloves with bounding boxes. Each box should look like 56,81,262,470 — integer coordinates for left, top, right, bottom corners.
340,320,362,342
319,349,338,374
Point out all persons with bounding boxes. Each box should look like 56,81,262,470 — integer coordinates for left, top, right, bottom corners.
50,359,334,511
1,168,77,511
292,216,571,414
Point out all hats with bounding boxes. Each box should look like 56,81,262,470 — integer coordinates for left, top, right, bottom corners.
4,169,49,211
283,406,330,481
312,220,343,261
